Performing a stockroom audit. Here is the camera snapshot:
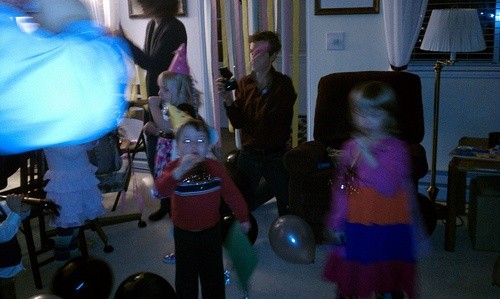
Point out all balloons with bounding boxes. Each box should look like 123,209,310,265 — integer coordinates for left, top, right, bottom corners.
225,149,243,173
136,173,162,208
114,272,176,299
222,214,258,251
268,214,316,264
51,256,112,299
28,293,63,299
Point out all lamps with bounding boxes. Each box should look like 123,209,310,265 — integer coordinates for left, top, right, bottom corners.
420,9,487,220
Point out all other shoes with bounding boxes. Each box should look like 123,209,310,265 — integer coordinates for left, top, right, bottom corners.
163,253,176,263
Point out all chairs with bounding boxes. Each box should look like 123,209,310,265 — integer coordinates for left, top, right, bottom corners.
0,106,152,289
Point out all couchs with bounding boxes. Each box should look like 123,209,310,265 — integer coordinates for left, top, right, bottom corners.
283,72,428,242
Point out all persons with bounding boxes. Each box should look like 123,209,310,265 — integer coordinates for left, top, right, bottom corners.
43,144,114,258
0,193,31,299
154,102,251,299
111,0,187,223
322,80,420,299
0,0,136,154
215,30,298,215
141,43,203,265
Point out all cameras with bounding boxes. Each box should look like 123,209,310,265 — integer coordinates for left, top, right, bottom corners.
223,78,238,91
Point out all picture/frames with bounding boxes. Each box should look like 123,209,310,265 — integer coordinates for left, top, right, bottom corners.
315,0,380,16
128,0,187,18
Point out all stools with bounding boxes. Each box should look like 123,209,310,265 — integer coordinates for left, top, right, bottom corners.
468,174,500,251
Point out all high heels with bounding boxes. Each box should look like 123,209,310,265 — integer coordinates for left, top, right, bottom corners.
149,200,171,222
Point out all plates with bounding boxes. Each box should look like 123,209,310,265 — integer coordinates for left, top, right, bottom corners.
456,145,473,152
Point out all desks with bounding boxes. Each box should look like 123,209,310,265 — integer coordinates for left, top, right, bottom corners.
445,137,500,252
